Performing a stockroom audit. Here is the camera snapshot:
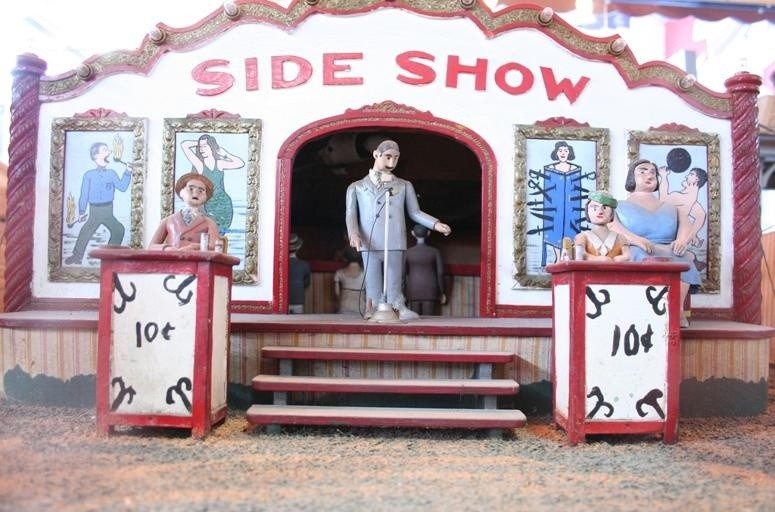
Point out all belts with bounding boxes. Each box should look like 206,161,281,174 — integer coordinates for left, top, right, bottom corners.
88,199,113,208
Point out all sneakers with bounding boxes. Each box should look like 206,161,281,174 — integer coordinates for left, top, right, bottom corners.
64,253,82,265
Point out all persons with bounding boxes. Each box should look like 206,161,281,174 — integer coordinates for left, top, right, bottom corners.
344,138,452,320
610,158,705,329
148,171,221,254
333,241,367,315
658,148,709,258
287,232,314,313
180,135,245,240
540,137,582,270
64,142,133,266
571,190,632,262
404,223,447,316
